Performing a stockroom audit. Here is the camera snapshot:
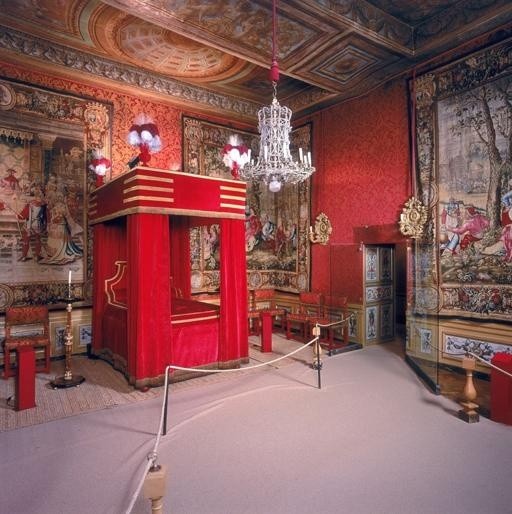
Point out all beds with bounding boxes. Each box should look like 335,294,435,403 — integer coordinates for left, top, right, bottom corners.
91,164,249,390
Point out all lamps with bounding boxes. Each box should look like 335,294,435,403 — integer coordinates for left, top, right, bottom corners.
240,0,316,193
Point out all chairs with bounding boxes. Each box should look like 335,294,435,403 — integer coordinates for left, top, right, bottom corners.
4,305,50,379
253,289,286,333
249,291,260,336
489,353,512,426
309,294,348,351
286,292,320,344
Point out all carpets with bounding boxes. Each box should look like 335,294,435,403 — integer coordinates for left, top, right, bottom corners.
0,328,325,431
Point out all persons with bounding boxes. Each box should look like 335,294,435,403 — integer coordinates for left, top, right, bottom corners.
0,167,84,267
0,130,39,149
438,190,512,264
205,206,298,270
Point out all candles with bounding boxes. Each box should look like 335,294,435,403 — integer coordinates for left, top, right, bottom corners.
69,270,71,284
